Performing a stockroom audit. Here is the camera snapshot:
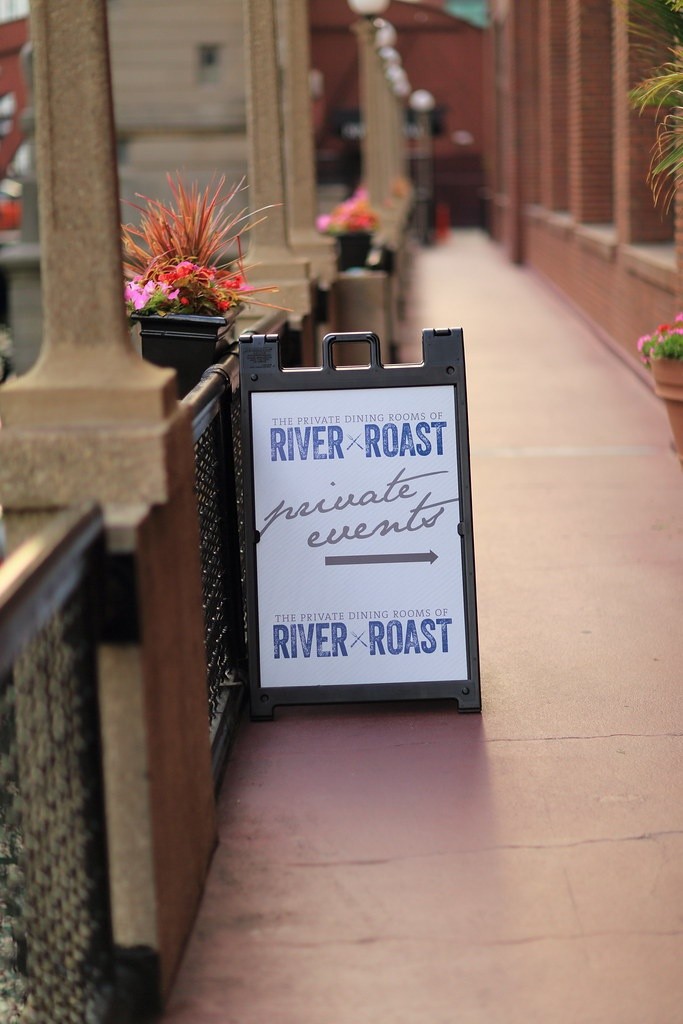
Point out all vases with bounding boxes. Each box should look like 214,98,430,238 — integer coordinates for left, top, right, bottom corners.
652,357,683,469
336,234,374,268
129,306,245,372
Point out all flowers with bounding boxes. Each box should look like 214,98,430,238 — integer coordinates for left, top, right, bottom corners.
113,185,295,315
631,314,683,367
314,188,383,233
